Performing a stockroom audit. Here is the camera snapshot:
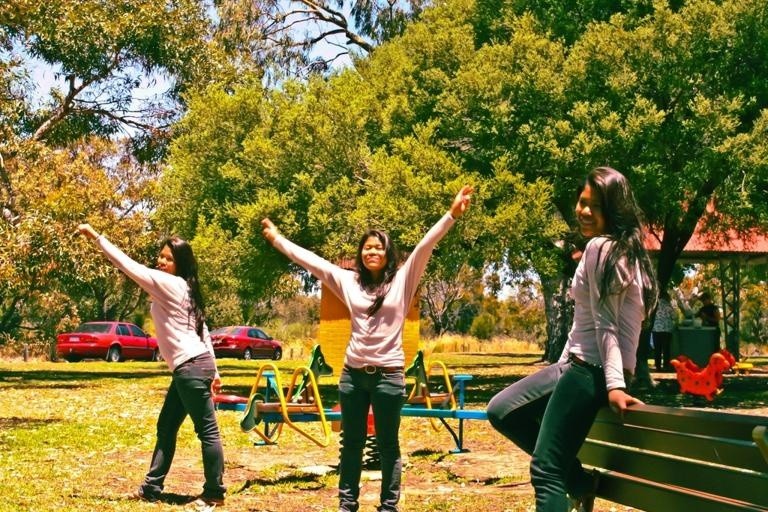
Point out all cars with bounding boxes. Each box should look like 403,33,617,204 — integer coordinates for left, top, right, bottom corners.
209,326,283,361
58,321,159,362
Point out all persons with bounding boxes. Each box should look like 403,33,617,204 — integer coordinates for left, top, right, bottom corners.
654,288,675,369
257,185,474,512
693,292,722,351
487,165,653,512
76,221,228,505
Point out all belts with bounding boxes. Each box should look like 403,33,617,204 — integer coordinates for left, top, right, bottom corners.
567,351,603,374
361,365,395,374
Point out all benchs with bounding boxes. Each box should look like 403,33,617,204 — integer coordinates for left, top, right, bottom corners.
581,400,767,512
